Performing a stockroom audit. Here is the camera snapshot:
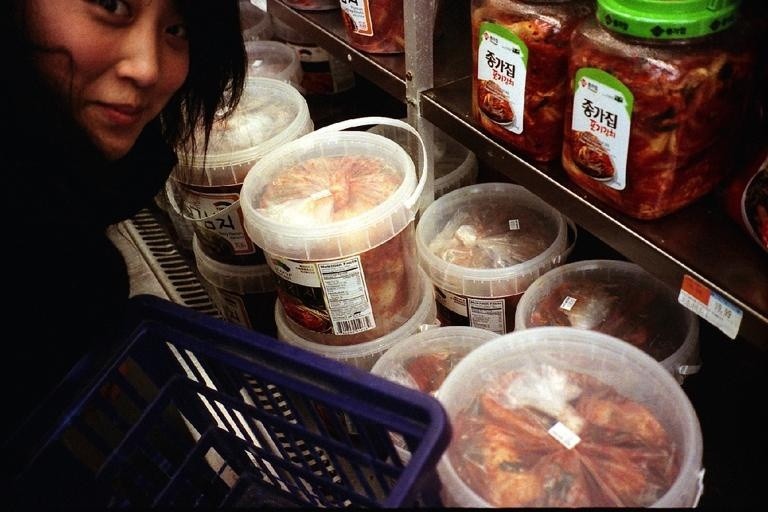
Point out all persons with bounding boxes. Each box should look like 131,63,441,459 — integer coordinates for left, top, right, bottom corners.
0,0,248,512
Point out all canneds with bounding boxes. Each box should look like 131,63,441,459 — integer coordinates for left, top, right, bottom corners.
280,0,403,55
561,1,754,221
470,0,591,161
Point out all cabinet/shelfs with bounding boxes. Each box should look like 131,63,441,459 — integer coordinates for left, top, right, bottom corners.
111,0,767,510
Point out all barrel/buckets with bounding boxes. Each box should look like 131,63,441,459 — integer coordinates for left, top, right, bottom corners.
162,38,709,509
